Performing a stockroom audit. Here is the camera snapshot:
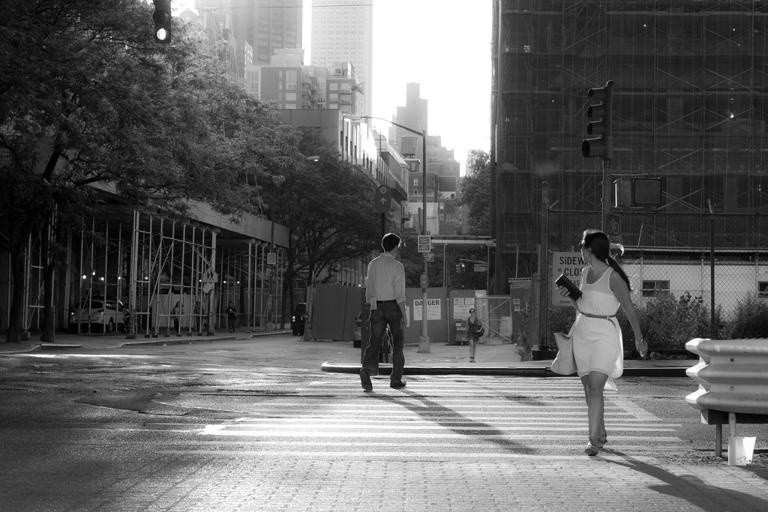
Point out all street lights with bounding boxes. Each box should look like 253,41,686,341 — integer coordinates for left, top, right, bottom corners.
340,113,430,355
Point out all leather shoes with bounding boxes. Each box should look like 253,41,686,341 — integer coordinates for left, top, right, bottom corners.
360,367,372,390
390,381,406,388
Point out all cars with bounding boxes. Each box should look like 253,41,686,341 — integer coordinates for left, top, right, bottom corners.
352,312,392,348
290,302,306,336
69,299,129,333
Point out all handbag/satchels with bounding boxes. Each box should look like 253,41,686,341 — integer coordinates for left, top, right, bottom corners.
555,274,582,301
550,331,576,375
478,325,484,336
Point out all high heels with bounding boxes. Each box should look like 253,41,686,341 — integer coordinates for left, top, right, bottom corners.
584,430,606,456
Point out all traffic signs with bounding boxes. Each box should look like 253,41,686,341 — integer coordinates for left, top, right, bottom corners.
417,235,430,253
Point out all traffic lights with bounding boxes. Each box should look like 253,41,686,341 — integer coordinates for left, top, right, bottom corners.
152,0,170,43
455,264,459,272
581,87,606,158
459,263,464,273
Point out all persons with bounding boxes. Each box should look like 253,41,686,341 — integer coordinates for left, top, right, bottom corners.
466,307,479,363
556,228,648,456
359,231,407,390
120,300,238,336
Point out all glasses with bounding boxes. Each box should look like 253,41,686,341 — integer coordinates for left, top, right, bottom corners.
469,310,474,313
578,243,583,249
